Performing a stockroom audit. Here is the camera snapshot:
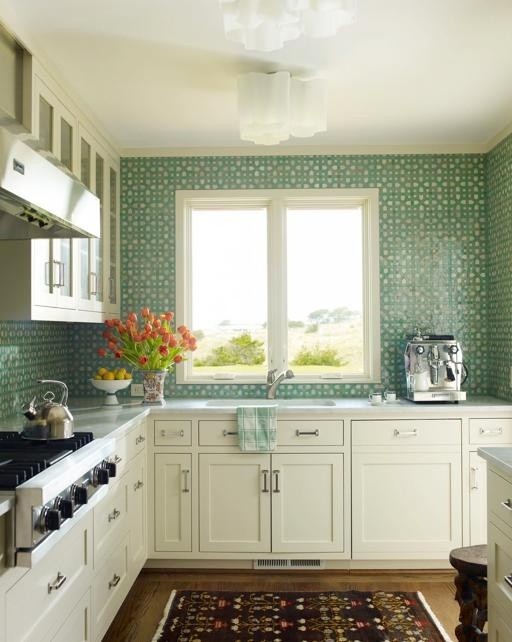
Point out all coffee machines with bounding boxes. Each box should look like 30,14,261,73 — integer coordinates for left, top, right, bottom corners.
403,338,467,402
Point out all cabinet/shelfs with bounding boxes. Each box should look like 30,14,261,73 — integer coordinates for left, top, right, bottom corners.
146,409,196,567
2,53,123,324
352,409,464,570
0,416,150,642
198,409,352,569
476,448,512,642
465,406,511,546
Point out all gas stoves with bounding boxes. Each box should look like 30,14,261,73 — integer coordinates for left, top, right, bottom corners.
0,430,121,548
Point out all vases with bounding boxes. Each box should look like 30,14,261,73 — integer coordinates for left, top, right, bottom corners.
133,369,166,406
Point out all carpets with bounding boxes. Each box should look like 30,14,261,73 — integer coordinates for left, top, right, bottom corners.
151,588,452,642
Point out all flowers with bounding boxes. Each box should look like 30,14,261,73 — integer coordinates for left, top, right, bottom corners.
96,308,198,371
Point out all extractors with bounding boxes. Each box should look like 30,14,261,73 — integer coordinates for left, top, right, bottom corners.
0,106,101,239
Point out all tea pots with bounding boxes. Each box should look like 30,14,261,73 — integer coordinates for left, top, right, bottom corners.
20,379,75,442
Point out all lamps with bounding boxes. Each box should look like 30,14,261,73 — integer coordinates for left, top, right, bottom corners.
220,69,355,148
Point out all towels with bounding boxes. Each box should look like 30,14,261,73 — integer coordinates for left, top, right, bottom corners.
236,404,278,452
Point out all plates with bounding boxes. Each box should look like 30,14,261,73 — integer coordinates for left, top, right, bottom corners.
383,399,401,404
367,402,385,406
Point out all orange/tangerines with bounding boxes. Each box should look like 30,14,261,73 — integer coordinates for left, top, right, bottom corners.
94,367,133,381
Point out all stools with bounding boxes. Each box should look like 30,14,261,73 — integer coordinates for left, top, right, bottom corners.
449,545,488,642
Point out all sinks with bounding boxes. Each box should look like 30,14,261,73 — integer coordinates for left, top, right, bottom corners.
205,399,337,408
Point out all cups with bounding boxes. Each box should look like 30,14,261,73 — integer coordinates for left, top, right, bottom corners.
368,393,383,402
384,391,397,401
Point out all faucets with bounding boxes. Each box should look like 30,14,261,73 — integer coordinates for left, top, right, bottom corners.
268,369,295,399
